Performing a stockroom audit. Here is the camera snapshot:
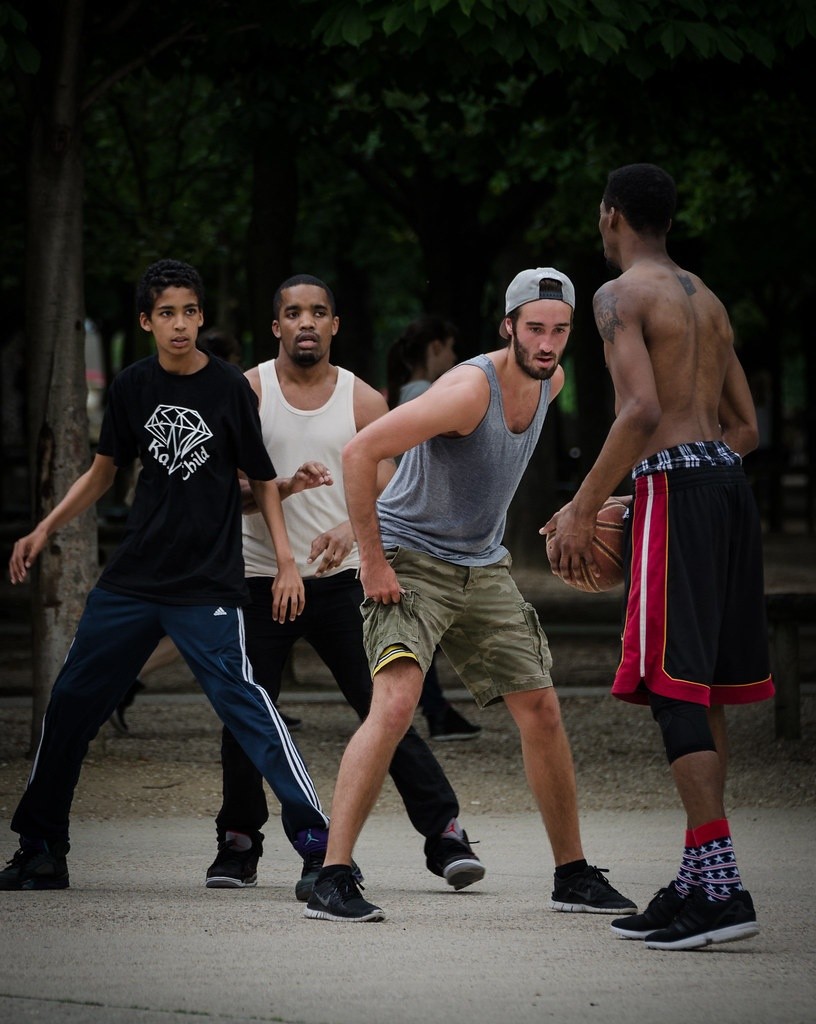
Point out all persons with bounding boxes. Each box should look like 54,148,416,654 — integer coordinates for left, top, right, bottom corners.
378,319,488,744
0,259,369,908
532,159,780,957
292,264,640,928
103,632,303,746
200,274,488,897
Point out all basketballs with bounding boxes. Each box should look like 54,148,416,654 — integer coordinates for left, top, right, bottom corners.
545,497,631,594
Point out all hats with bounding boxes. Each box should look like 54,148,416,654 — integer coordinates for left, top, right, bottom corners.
504,266,575,316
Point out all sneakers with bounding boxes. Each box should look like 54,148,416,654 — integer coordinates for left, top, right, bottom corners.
295,827,363,901
431,817,486,891
205,829,259,888
609,880,761,950
304,870,388,924
428,710,484,742
547,864,639,916
